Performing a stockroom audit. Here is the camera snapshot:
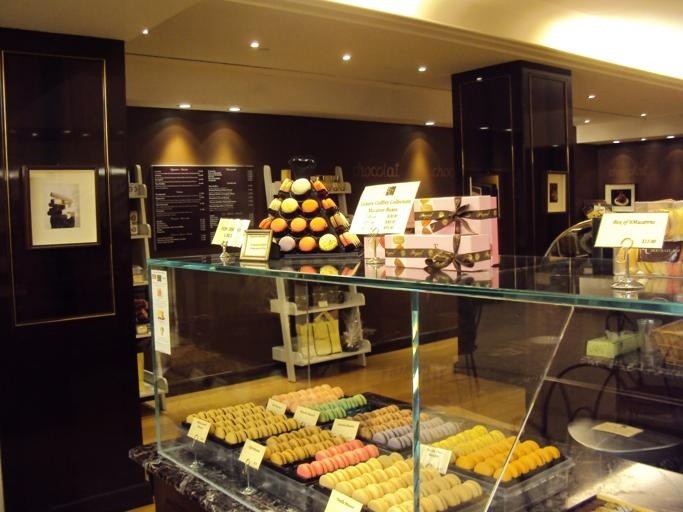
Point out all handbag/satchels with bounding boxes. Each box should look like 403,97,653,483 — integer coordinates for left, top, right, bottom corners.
296,320,342,359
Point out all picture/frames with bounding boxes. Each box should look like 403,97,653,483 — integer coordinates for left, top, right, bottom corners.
544,169,568,215
22,164,105,250
603,183,636,214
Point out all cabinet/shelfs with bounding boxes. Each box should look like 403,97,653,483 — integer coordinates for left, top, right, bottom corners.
128,164,168,411
263,164,372,381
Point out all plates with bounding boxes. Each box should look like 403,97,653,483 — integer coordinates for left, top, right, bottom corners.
529,336,558,344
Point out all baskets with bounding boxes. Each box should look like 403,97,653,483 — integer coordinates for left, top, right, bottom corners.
651,319,682,368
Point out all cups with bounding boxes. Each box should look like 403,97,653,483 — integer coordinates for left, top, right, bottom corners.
636,318,663,353
